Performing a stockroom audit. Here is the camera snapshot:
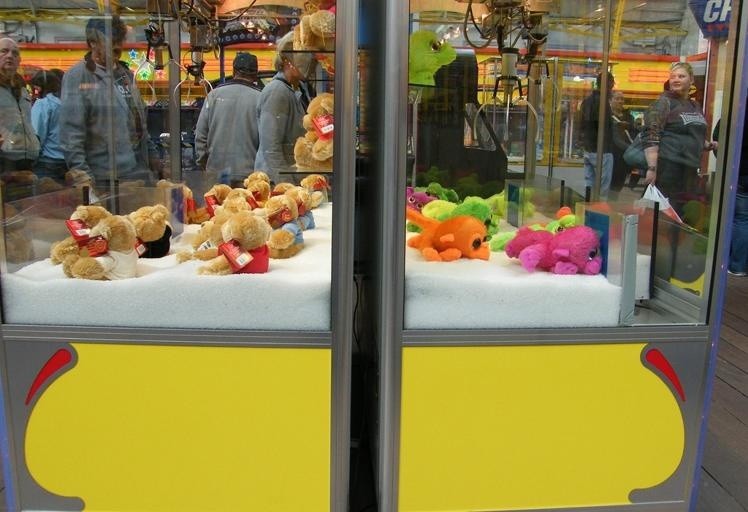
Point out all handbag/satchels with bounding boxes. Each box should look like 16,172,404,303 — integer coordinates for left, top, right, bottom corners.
623,134,647,170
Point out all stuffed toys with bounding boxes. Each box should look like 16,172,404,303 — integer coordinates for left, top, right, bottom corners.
408,28,458,89
294,92,334,171
407,182,602,274
51,172,327,279
49,90,607,283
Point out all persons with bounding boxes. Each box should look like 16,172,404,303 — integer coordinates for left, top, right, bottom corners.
255,33,322,187
1,38,42,212
192,52,267,186
26,68,69,184
61,15,164,211
576,62,748,278
